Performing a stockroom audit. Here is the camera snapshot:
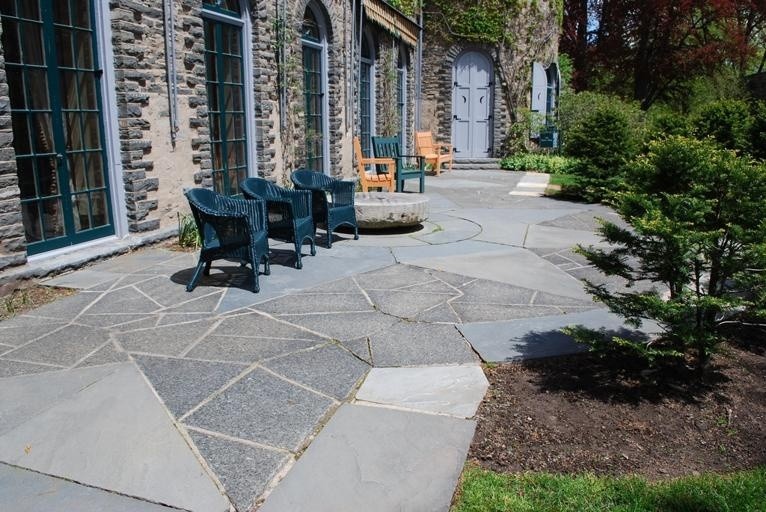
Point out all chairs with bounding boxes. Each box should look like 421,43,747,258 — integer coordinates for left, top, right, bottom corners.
184,188,270,293
241,178,315,269
290,170,359,248
354,137,395,192
372,136,425,192
416,131,452,176
